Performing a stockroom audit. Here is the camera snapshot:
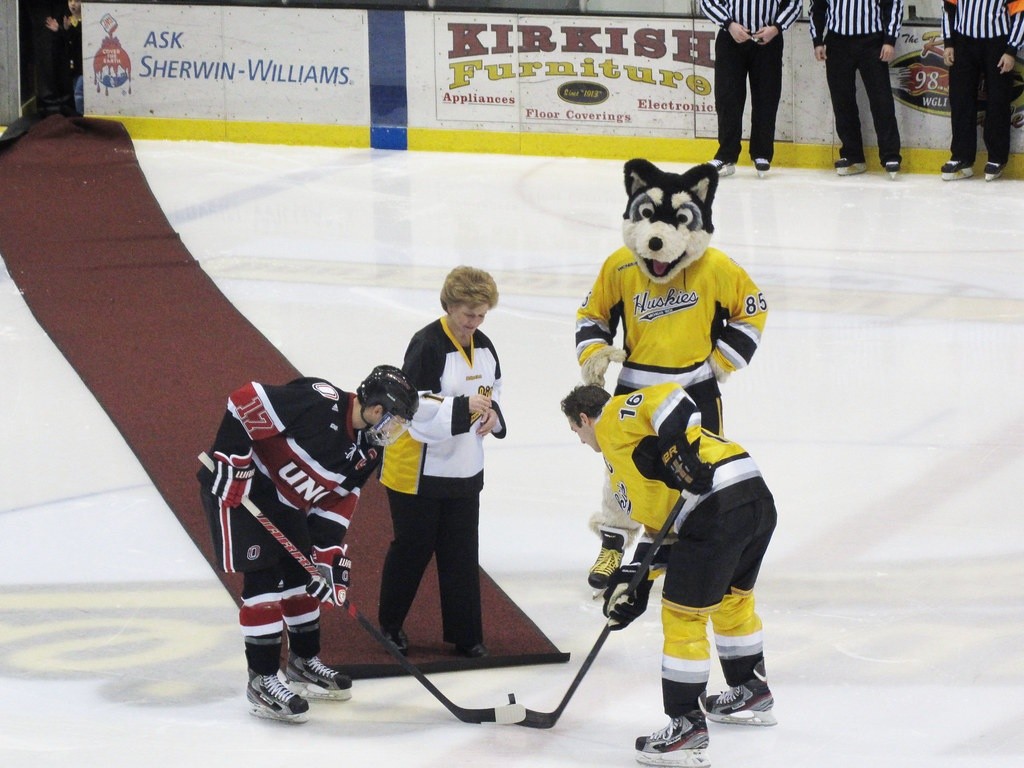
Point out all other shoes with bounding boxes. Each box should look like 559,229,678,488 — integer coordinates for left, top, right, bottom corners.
72,113,93,130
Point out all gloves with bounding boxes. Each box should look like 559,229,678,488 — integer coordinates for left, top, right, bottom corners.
651,431,715,496
306,544,352,606
209,446,253,509
603,563,655,631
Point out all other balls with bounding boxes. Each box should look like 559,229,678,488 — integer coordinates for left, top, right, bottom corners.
508,693,515,704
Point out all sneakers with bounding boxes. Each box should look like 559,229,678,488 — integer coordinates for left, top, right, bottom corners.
284,646,353,700
940,161,974,180
634,709,711,768
755,157,771,178
834,158,867,176
885,160,900,179
983,160,1007,181
706,159,736,176
703,677,779,726
245,664,310,723
587,524,628,599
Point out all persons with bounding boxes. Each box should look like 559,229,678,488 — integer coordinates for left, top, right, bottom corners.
198,366,420,727
693,1,806,178
378,268,508,662
557,385,781,768
808,1,904,180
44,0,82,115
939,1,1024,180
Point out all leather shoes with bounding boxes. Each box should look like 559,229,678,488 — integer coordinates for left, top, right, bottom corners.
451,641,489,659
379,621,409,655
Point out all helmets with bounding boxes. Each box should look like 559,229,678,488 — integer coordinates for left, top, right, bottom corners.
361,364,420,421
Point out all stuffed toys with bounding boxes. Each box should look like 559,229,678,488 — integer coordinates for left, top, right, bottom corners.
572,157,768,601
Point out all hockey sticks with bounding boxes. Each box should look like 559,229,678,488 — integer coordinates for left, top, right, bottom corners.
197,451,527,723
518,461,716,729
